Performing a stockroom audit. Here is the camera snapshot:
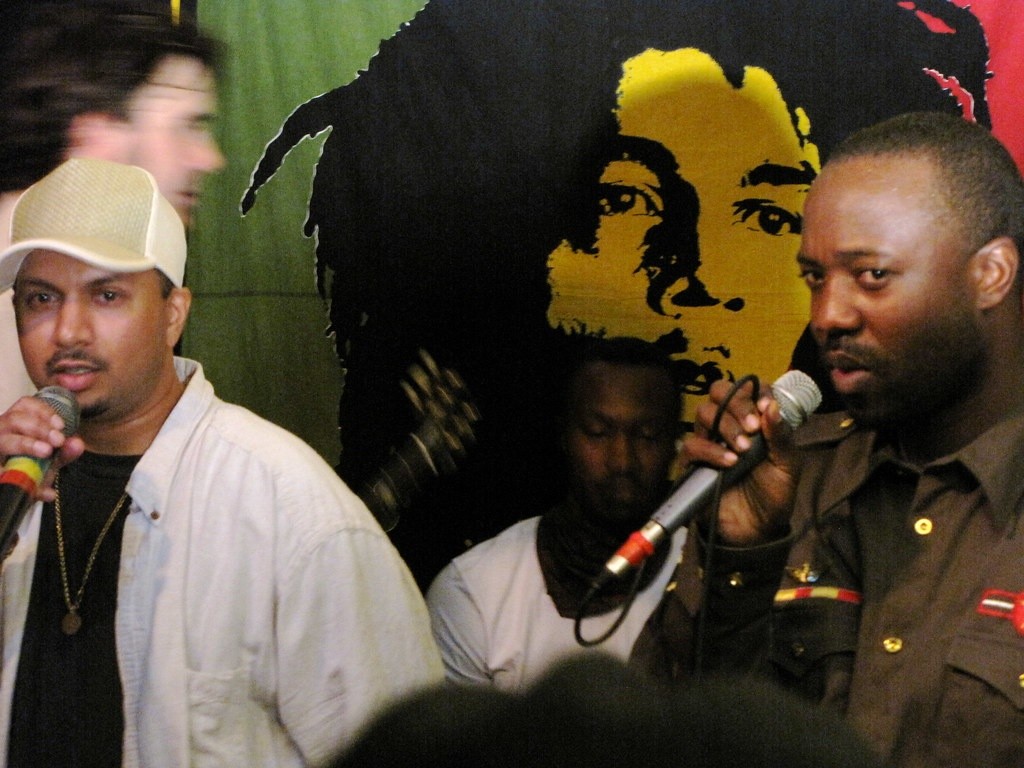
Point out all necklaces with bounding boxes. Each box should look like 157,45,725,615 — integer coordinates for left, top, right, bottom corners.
55,470,128,634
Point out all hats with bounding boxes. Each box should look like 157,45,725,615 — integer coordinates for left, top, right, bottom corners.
0,158,189,294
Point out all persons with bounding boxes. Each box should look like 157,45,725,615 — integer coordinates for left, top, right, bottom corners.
0,158,443,768
0,17,228,415
425,334,687,694
630,114,1024,767
320,652,882,768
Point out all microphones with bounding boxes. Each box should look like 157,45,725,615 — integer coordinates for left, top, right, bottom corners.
1,384,82,552
583,368,824,601
